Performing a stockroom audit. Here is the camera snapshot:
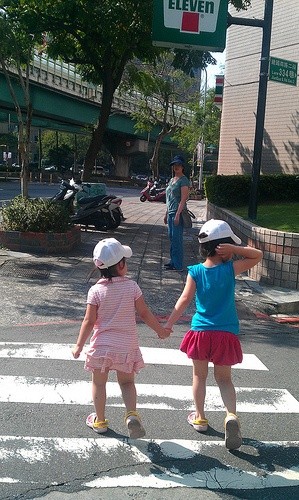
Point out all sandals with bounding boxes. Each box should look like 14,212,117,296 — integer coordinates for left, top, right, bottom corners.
86,412,110,433
224,413,243,450
187,411,208,432
124,410,146,439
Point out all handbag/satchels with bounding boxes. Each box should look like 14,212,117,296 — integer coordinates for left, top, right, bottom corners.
182,210,195,228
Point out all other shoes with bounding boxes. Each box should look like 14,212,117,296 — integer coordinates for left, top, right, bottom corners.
164,263,174,267
166,266,184,272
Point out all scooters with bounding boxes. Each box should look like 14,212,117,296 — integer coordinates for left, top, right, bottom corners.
52,166,126,231
140,180,168,203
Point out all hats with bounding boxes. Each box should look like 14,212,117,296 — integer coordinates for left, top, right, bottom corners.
197,218,241,245
169,155,186,167
92,237,132,270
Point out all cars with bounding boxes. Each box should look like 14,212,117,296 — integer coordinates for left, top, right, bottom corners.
136,175,145,180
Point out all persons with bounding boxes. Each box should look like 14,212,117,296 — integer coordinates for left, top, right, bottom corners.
165,156,189,271
71,238,174,439
155,219,263,451
